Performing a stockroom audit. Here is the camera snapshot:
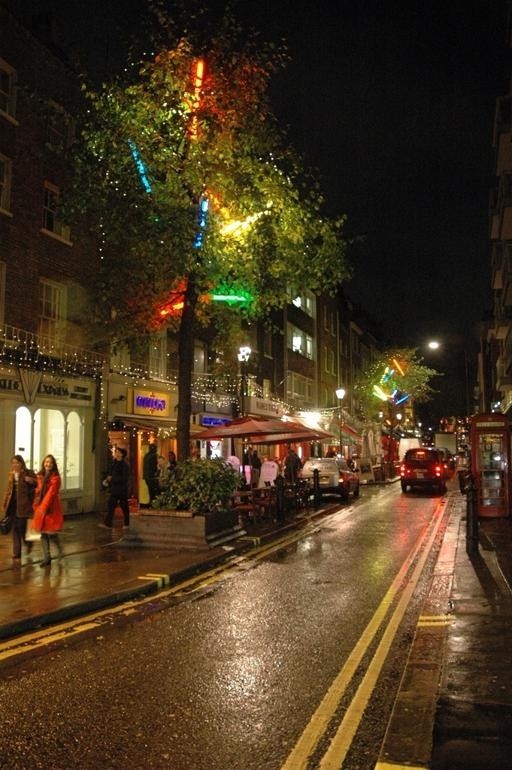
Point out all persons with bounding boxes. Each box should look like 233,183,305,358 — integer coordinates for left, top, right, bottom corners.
98,447,133,530
3,454,66,567
242,447,302,483
142,444,177,507
9,564,63,593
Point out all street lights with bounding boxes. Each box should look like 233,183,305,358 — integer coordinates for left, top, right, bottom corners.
336,389,345,458
237,346,251,503
416,342,440,350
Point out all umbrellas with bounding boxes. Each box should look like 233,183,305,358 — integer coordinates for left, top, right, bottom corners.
189,415,335,447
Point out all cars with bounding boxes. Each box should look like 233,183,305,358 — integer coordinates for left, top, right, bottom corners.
400,447,455,495
301,458,359,502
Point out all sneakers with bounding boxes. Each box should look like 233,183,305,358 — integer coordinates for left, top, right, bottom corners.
40,560,51,568
98,522,113,530
123,523,130,529
56,550,63,562
11,556,22,570
27,547,33,554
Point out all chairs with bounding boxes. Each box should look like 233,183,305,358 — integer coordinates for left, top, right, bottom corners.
235,479,310,539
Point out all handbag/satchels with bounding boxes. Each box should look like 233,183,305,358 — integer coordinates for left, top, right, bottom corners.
0,514,14,535
24,519,42,542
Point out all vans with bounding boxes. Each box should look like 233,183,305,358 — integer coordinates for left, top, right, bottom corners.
398,438,423,463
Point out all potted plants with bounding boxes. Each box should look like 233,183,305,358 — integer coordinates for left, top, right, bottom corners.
120,456,238,548
